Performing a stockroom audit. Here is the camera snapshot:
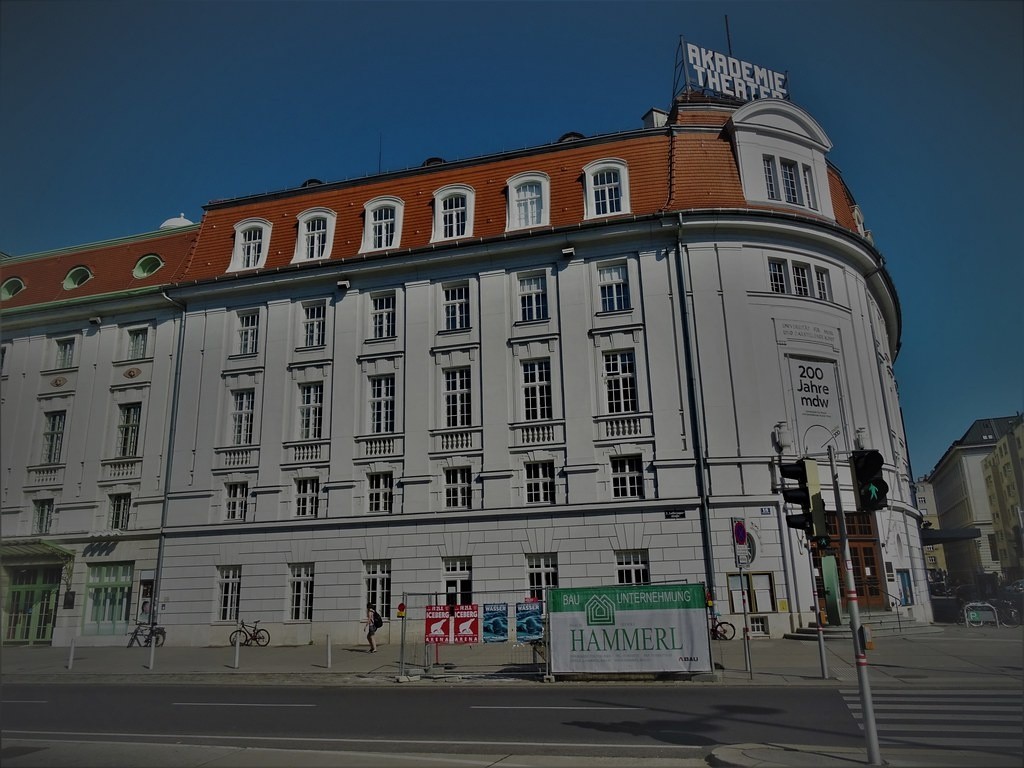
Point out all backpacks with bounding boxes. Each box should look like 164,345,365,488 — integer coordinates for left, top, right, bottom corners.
368,610,382,628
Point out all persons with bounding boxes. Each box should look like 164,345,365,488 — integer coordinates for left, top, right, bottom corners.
55,378,62,386
128,369,136,377
364,602,378,653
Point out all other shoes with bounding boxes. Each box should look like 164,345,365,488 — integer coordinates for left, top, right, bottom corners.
366,649,378,654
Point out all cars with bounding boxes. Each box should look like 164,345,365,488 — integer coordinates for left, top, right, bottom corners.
955,585,978,596
932,583,945,595
1001,579,1024,610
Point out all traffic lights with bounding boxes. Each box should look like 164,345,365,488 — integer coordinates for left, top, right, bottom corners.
848,449,889,513
778,459,826,539
817,535,830,550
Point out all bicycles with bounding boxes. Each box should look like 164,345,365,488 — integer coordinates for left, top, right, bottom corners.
127,618,166,648
955,596,1021,629
708,612,735,641
229,619,271,647
